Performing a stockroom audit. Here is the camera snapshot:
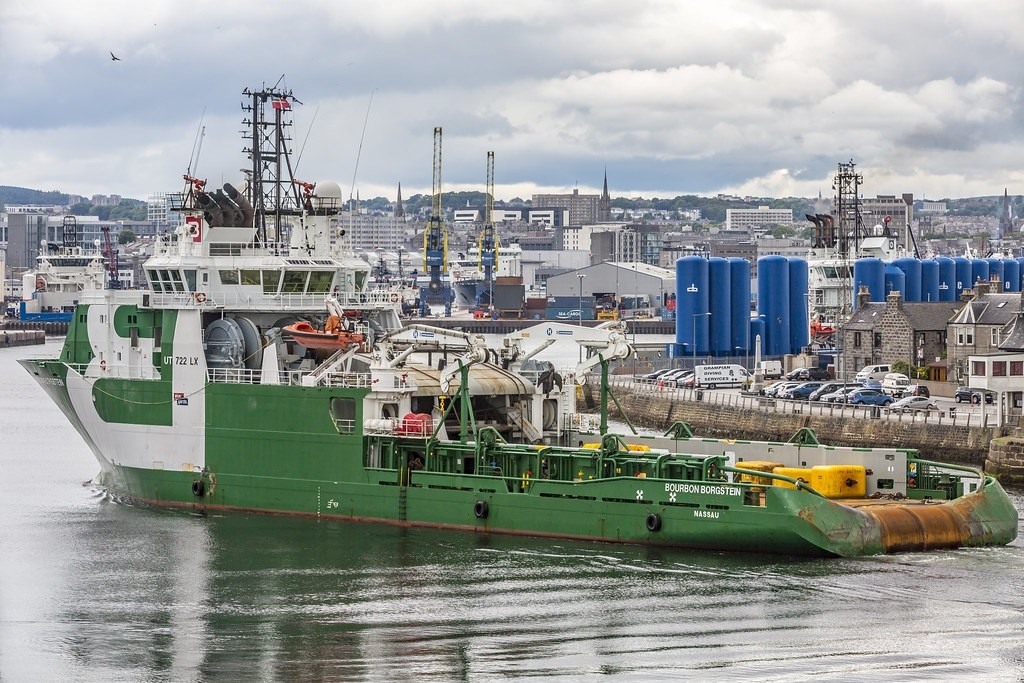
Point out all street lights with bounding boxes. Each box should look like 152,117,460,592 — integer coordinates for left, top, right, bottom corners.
776,316,783,360
576,274,587,326
745,313,765,370
692,312,712,369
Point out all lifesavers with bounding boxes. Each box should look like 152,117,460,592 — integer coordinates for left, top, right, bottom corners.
196,292,207,303
192,480,205,497
390,294,399,303
646,512,661,532
474,500,489,519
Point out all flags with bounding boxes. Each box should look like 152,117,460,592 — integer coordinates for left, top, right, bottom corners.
272,98,290,109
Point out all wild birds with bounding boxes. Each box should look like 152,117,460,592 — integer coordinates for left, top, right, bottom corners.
110,50,122,61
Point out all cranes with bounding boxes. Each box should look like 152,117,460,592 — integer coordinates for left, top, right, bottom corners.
419,126,456,317
475,150,504,317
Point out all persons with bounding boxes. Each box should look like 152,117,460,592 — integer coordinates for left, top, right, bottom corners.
790,477,810,495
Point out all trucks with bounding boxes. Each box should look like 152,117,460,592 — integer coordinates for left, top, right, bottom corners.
761,361,781,379
695,364,753,389
852,365,892,380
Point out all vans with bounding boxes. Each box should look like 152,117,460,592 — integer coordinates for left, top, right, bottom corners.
881,373,909,397
809,383,863,401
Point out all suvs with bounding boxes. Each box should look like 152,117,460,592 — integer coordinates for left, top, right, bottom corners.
902,385,930,399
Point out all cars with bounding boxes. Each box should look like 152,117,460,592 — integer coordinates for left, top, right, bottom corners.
820,387,895,408
642,369,694,387
762,381,829,399
786,367,831,381
889,396,939,412
854,377,882,392
955,386,993,404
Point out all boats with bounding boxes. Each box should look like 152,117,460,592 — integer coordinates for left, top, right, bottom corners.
15,73,1018,559
